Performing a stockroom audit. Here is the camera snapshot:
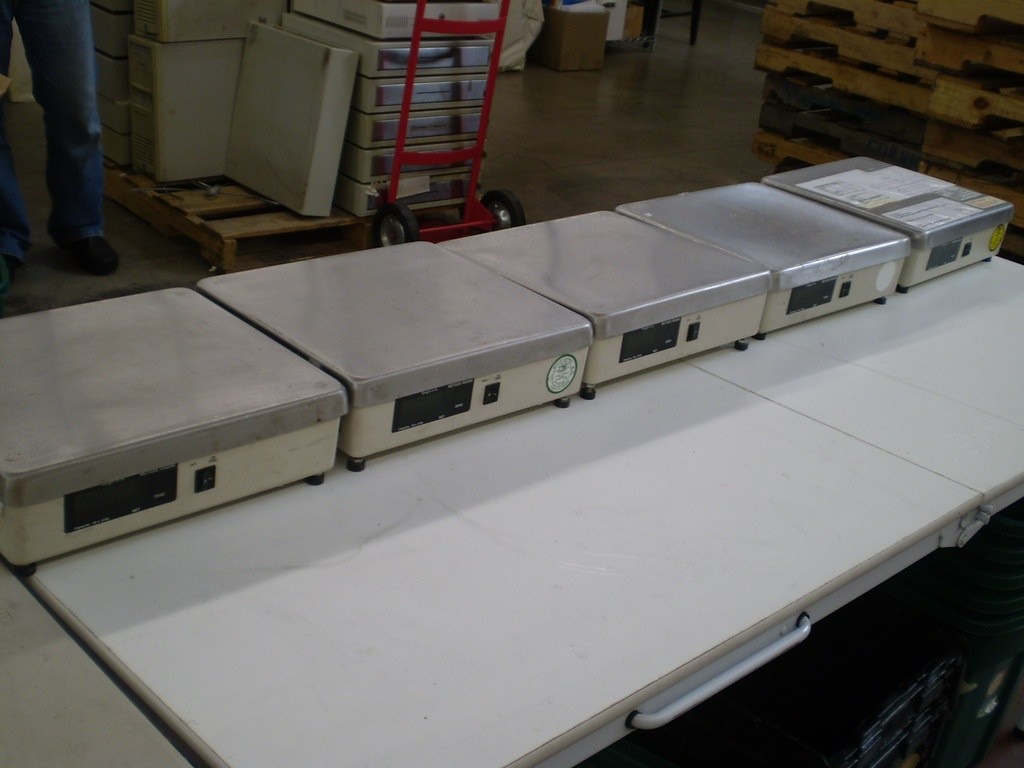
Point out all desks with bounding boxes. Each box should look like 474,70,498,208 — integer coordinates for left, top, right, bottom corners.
17,256,1024,768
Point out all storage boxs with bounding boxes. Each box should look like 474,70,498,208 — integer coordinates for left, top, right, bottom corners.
578,498,1024,768
532,3,607,71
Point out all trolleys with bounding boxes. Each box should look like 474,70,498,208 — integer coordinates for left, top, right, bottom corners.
366,0,527,249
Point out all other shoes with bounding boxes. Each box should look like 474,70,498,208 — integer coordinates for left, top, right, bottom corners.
4,254,18,281
74,234,119,275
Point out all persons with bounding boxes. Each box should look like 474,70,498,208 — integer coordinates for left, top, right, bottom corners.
0,0,117,293
